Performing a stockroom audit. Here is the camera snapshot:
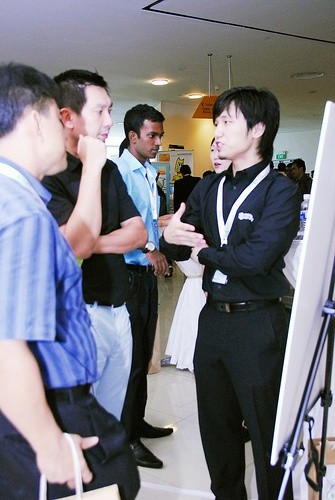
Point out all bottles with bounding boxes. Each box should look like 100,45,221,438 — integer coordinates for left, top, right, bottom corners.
299,194,310,233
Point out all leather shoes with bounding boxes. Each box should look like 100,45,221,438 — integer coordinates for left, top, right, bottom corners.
137,420,173,438
129,439,163,469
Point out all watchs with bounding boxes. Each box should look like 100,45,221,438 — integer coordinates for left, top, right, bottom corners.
142,242,156,253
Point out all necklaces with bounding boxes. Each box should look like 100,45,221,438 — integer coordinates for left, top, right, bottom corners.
1,164,46,207
212,164,270,285
139,168,160,248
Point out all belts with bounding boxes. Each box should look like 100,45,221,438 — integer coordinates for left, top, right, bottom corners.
127,264,155,273
207,297,282,313
45,383,92,402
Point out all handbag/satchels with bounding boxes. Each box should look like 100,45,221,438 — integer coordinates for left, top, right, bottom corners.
39,433,121,500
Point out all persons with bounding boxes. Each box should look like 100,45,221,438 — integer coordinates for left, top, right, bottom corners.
202,137,232,298
163,85,302,500
119,139,216,280
115,103,171,469
0,62,141,500
270,158,314,194
41,69,148,422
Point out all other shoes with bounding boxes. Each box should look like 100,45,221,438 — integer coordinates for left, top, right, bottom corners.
164,266,174,277
